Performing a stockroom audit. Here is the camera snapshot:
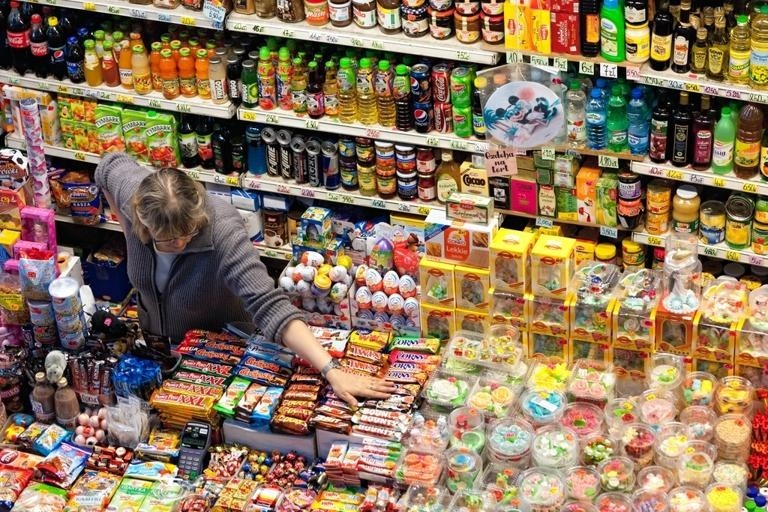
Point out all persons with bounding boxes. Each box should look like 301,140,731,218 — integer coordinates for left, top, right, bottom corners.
94,153,396,407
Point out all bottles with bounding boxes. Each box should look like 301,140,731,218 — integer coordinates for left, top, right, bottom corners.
31,372,81,429
0,0,768,188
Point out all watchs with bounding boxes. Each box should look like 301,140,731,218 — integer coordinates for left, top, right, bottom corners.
320,357,339,379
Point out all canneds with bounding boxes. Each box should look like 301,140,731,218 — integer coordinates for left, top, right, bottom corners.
699,191,757,250
616,168,642,201
645,179,671,234
303,1,504,44
260,126,440,202
410,56,475,138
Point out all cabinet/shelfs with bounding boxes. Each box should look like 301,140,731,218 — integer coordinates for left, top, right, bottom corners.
0,0,768,268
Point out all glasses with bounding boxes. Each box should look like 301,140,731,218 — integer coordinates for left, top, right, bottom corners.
153,228,200,244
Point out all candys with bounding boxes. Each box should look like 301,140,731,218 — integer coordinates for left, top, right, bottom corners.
204,443,305,489
